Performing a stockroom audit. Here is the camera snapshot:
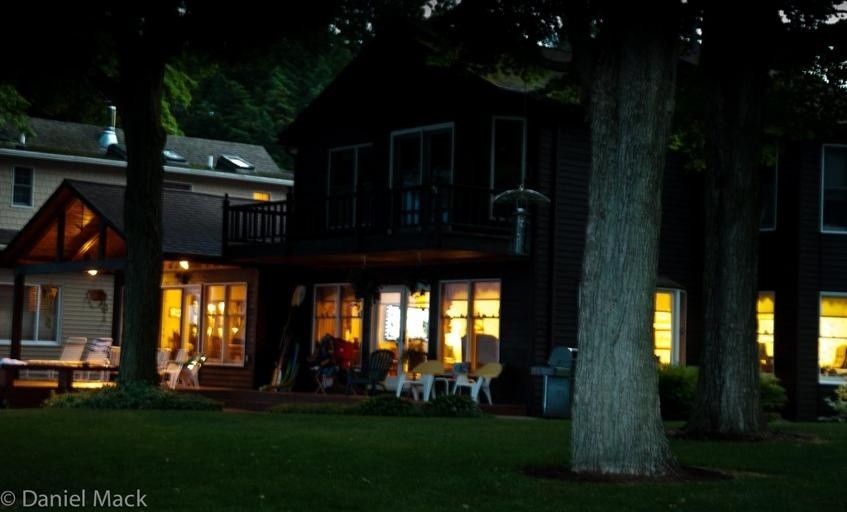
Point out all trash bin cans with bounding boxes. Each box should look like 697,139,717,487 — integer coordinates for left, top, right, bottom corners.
543,346,578,419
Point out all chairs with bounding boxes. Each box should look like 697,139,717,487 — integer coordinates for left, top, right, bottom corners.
396,361,507,404
26,335,203,388
348,350,393,395
